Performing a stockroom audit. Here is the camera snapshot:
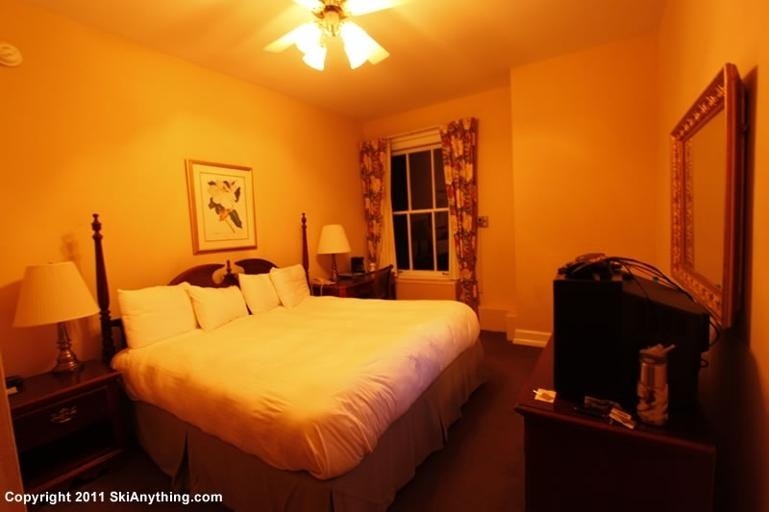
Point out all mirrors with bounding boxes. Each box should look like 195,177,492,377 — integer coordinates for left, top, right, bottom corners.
670,62,746,330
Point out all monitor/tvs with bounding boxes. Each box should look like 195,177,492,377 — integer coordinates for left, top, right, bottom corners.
552,257,712,409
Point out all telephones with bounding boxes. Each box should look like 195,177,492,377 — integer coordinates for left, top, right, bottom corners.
312,277,334,286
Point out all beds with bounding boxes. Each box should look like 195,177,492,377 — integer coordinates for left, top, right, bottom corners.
92,214,486,511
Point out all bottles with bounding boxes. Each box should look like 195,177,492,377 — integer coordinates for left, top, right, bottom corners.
637,344,669,427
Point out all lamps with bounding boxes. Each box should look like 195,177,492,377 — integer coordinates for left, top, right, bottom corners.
263,0,391,70
317,224,352,283
13,261,101,376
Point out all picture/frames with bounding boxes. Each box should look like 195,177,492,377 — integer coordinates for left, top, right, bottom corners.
185,158,257,255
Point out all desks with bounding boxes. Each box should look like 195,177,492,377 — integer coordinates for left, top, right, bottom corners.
514,331,716,512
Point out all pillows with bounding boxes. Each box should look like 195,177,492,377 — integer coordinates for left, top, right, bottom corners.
184,285,249,333
239,273,282,315
117,281,200,353
269,264,310,309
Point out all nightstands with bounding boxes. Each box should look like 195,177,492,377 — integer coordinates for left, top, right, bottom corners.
7,359,124,494
311,264,393,299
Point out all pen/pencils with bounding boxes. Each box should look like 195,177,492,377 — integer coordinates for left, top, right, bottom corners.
574,406,606,419
